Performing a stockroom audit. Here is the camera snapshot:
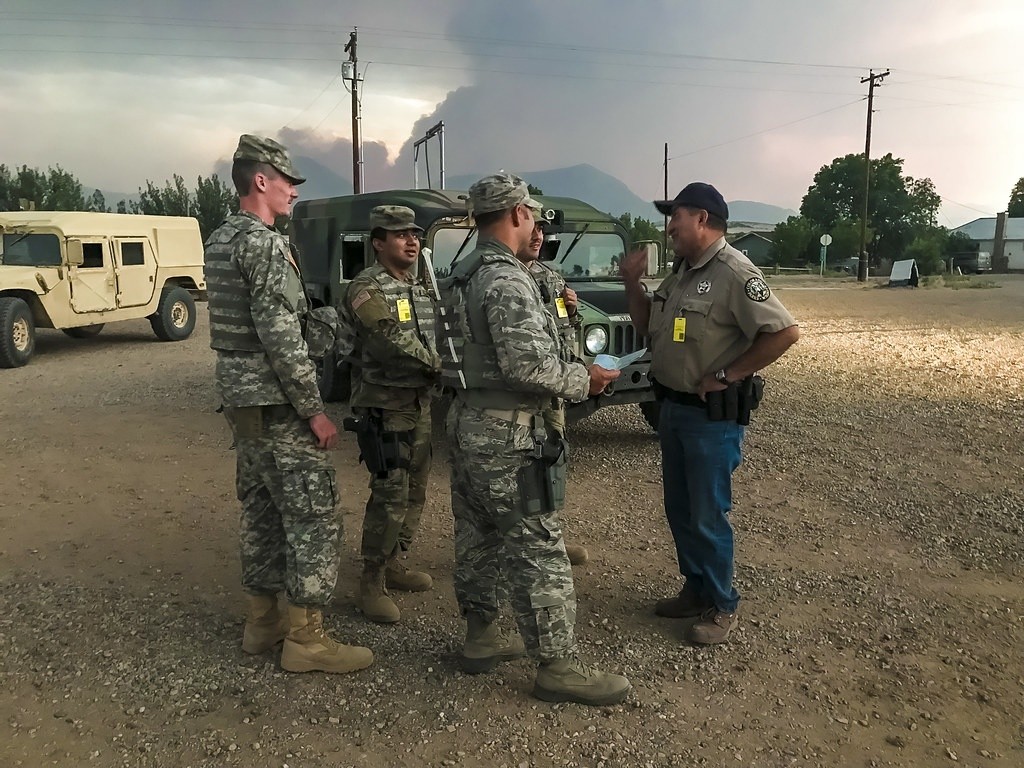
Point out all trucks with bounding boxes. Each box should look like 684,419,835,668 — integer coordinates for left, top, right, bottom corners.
289,188,659,435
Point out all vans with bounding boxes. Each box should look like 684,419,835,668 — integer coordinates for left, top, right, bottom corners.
946,250,993,275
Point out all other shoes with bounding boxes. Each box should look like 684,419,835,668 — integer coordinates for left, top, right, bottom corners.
565,545,588,563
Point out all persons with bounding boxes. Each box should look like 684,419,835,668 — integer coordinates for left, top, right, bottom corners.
518,206,589,567
203,135,374,673
434,172,631,706
619,183,800,645
340,204,443,622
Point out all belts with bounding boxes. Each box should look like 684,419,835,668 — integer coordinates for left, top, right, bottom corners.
660,384,706,408
474,407,534,428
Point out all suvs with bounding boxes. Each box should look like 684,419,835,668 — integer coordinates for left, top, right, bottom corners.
0,210,207,370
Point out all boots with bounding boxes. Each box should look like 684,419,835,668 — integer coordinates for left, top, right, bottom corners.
281,604,374,673
461,610,527,674
385,553,433,592
242,594,291,655
533,651,631,706
689,602,738,643
356,567,400,622
656,575,715,617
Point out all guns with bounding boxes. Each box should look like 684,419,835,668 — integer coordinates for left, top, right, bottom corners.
738,373,756,426
535,436,571,510
341,414,390,481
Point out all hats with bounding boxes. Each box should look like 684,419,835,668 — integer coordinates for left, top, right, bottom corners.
653,182,729,219
233,134,306,185
369,205,425,233
531,207,551,226
469,174,543,217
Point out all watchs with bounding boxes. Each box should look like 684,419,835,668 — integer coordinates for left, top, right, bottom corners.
716,368,731,386
